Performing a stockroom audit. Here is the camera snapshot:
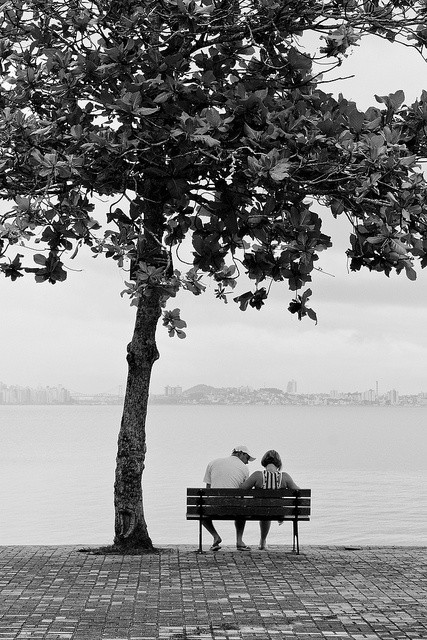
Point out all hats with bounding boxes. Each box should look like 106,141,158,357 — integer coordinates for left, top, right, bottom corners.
231,446,256,461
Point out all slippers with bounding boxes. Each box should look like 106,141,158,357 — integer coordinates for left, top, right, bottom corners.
237,546,251,551
209,545,221,551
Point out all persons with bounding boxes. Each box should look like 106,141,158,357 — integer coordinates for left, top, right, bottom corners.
241,450,300,550
203,445,256,551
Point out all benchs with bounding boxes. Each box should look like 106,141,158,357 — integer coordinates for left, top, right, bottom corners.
187,487,311,556
